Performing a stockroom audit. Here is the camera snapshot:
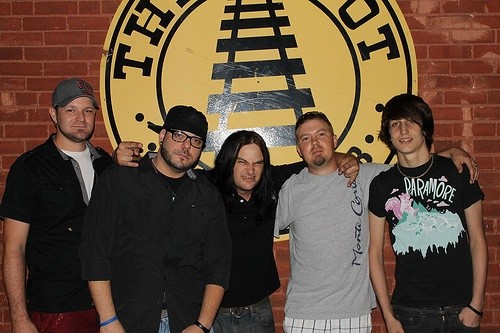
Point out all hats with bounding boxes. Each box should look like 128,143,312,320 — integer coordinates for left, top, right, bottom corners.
52,79,100,109
147,105,208,140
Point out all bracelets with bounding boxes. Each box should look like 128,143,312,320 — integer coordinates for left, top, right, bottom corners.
466,304,481,317
195,320,210,333
98,316,118,327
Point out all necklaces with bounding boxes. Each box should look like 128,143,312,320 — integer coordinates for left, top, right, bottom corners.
397,154,434,178
152,161,176,202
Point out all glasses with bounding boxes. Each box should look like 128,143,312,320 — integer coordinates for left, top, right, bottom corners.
167,129,204,149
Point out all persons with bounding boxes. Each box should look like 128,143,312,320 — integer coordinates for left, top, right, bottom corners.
369,94,488,333
273,112,480,333
112,130,360,333
0,77,112,333
80,106,232,333
